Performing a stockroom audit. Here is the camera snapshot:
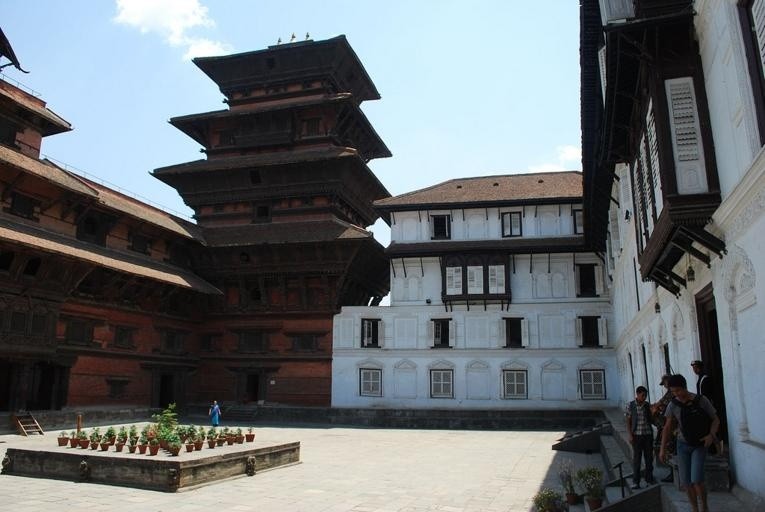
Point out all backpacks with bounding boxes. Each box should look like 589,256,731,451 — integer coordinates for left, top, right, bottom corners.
673,394,711,443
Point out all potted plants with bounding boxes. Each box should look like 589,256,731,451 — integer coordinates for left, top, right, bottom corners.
58,403,255,456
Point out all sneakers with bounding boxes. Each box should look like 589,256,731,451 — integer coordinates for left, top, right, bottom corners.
631,484,640,489
661,473,674,482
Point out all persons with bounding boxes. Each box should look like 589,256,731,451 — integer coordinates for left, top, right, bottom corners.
625,385,656,488
208,400,221,427
649,373,678,483
691,359,721,460
658,374,722,511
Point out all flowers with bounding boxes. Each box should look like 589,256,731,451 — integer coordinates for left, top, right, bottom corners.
532,465,604,512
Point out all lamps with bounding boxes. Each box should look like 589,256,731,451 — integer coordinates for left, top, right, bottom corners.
655,288,660,313
426,299,431,303
686,252,695,281
625,209,630,224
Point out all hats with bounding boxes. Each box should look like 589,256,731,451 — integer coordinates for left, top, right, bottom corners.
659,375,672,385
691,361,704,367
